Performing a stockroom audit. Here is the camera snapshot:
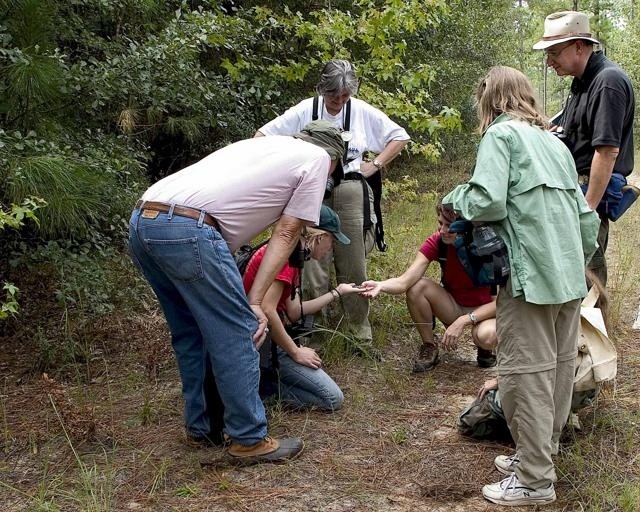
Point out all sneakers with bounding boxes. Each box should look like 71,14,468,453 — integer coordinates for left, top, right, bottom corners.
477,345,497,368
494,455,520,476
414,342,441,373
482,473,557,507
347,341,380,359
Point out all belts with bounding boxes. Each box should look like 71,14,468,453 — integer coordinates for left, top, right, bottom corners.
135,199,215,227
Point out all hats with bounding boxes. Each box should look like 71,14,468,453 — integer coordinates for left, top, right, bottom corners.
533,11,600,49
311,205,352,246
294,119,347,180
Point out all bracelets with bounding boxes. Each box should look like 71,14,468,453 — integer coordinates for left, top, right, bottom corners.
468,310,478,325
330,290,338,300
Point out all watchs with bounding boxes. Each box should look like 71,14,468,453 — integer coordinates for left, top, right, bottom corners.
372,159,381,169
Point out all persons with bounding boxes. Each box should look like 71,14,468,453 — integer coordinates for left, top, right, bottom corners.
128,120,349,467
532,11,635,290
241,205,373,413
456,269,617,447
254,58,411,345
357,193,500,373
441,65,602,508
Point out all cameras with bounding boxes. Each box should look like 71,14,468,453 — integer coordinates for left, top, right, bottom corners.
324,176,334,201
285,314,314,348
287,239,305,268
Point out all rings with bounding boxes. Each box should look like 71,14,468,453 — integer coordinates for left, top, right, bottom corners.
264,328,270,333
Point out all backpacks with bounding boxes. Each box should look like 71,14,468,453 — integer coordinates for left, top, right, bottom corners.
458,387,573,444
574,284,618,393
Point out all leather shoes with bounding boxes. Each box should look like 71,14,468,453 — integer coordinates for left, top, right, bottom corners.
227,436,306,467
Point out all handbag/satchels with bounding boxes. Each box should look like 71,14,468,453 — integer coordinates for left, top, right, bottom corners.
448,219,511,289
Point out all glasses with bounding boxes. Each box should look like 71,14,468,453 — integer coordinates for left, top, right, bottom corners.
543,43,576,58
